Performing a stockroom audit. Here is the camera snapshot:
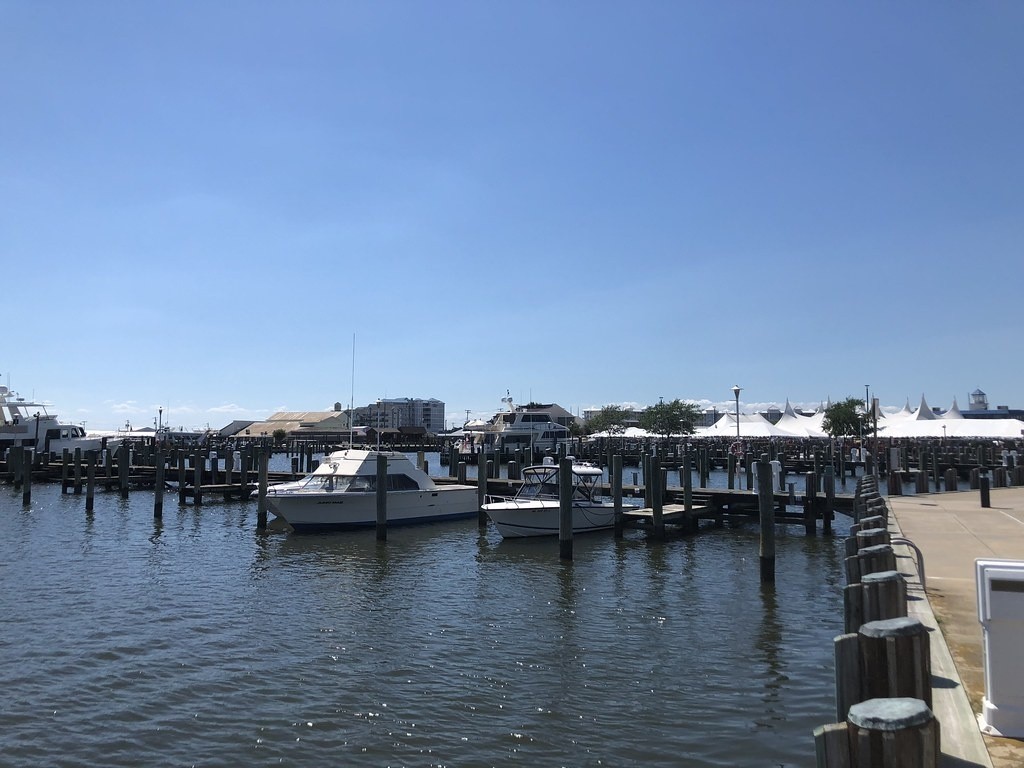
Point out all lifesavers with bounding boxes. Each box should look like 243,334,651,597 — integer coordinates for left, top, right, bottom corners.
731,442,747,457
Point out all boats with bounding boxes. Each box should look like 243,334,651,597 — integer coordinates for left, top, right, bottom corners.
268,331,482,529
249,479,300,519
0,383,105,462
480,462,640,538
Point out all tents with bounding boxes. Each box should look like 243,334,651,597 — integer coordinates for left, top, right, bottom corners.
586,413,1024,440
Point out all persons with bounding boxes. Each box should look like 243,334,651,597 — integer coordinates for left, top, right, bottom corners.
584,439,918,473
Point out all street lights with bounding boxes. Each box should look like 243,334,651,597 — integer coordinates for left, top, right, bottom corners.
374,397,382,451
865,383,870,419
158,407,163,436
732,384,744,442
941,423,948,448
